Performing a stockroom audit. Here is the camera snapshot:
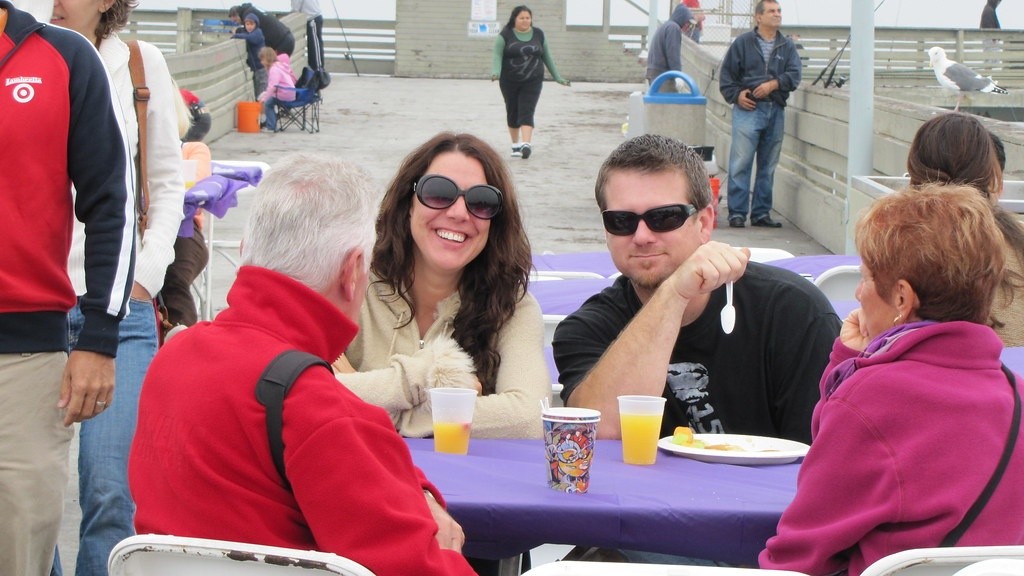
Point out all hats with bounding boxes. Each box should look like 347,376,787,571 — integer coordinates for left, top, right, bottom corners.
689,17,697,24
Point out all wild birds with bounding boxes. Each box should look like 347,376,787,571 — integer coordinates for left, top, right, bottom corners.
927,46,1008,111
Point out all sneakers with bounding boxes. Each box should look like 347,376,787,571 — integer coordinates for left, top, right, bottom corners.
511,142,522,157
520,144,531,159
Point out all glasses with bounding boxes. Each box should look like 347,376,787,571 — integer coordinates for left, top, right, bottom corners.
413,173,504,220
601,204,697,237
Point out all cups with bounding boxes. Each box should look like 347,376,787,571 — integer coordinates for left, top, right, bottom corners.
542,408,602,494
616,394,668,463
427,389,477,455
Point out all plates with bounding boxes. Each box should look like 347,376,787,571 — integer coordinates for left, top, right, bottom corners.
655,431,816,464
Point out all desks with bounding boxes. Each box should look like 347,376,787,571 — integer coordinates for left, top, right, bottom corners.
357,245,1023,565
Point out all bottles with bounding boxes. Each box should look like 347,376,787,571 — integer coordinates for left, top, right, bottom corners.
551,384,564,407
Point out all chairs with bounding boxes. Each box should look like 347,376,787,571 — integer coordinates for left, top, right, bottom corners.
268,65,321,135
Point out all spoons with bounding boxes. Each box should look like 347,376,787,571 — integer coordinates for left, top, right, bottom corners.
721,282,736,334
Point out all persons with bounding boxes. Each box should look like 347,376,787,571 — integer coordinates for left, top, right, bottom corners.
227,2,295,57
156,87,210,332
229,12,268,101
759,181,1022,576
680,0,706,41
904,111,1023,349
718,0,803,228
980,0,1002,69
261,46,296,133
550,133,842,444
127,152,482,576
256,47,297,103
0,0,130,576
38,0,186,576
330,130,551,441
645,3,696,95
291,0,324,72
491,5,571,159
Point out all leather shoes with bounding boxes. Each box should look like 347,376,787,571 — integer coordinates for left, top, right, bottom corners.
729,216,745,227
751,215,782,228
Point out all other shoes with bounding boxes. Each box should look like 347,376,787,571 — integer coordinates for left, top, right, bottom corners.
260,126,273,133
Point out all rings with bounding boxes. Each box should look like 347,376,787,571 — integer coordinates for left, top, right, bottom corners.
96,401,107,406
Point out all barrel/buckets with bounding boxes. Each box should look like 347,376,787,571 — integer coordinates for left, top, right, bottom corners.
236,102,263,133
